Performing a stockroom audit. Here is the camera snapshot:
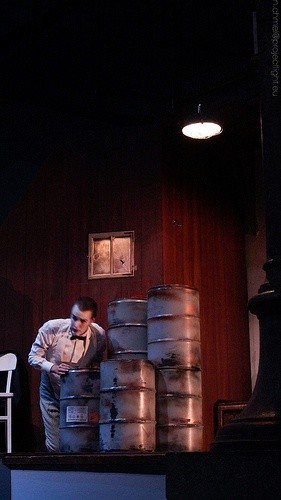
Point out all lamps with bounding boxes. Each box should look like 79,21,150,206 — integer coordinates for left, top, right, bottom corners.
181,96,224,140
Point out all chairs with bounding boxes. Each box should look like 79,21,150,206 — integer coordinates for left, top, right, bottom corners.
0,353,17,453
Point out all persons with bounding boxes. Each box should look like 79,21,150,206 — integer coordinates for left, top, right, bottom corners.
28,296,106,454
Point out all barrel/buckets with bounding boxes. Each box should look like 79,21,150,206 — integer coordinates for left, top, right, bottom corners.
106,298,149,358
100,357,155,452
145,285,202,370
154,367,204,453
58,368,100,451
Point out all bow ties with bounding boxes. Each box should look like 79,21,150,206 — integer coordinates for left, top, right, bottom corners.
70,335,86,340
